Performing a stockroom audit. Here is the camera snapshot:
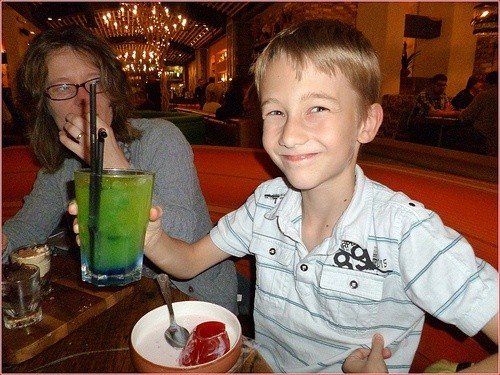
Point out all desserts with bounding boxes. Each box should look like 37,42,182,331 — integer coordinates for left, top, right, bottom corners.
177,321,230,367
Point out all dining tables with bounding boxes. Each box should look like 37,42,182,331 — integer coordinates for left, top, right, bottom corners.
0,238,271,375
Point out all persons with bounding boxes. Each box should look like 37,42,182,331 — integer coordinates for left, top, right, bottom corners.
1,25,239,316
342,334,392,373
203,78,260,119
452,75,483,110
67,18,499,374
141,81,161,110
407,75,455,142
194,77,215,105
457,71,498,156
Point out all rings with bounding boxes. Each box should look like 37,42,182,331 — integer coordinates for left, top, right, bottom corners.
76,132,86,140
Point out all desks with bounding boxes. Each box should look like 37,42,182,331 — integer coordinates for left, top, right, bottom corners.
422,115,459,146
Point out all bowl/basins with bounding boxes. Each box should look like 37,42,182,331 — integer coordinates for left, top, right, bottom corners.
130,300,242,373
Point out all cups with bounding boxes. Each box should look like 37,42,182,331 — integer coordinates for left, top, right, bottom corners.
2,264,42,329
74,168,155,286
8,244,52,296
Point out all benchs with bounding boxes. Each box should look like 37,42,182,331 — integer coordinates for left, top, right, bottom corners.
128,106,204,145
376,93,453,140
175,106,261,148
0,142,500,375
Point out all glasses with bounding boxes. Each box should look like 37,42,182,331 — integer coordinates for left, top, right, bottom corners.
43,77,110,101
437,83,448,86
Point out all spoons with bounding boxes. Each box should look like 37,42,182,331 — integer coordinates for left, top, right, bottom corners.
157,273,190,349
